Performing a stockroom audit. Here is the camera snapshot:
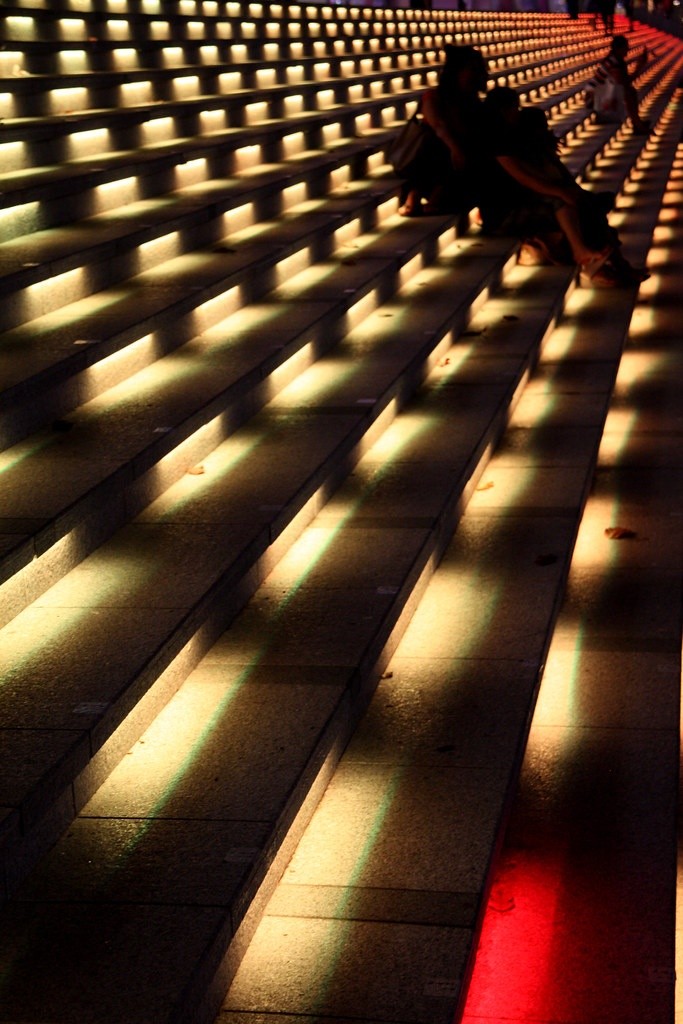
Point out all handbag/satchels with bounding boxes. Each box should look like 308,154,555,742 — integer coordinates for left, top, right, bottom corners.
387,92,440,178
593,76,626,126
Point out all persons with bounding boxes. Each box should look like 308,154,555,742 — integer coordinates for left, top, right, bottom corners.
600,0,617,37
584,35,654,136
621,0,642,32
388,45,651,289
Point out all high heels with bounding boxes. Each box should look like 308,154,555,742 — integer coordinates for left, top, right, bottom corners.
580,242,616,279
